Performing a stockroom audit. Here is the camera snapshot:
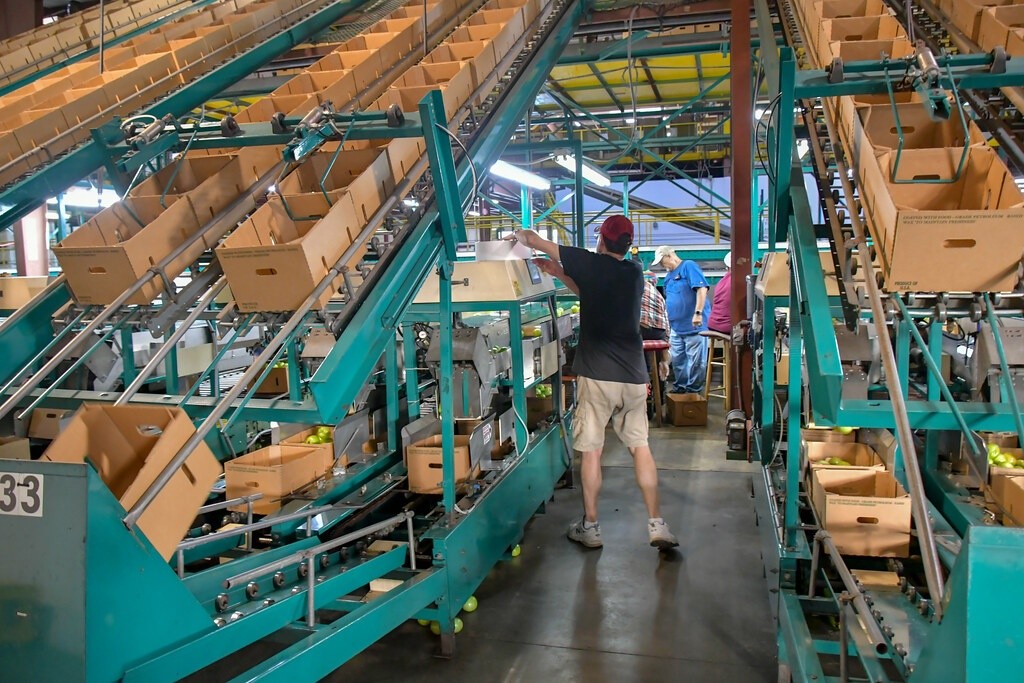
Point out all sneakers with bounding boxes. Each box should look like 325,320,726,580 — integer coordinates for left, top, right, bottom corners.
567,513,603,548
647,521,680,549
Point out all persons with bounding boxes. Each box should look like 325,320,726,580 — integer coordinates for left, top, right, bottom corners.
632,247,642,263
501,215,680,551
651,245,712,395
707,249,731,336
640,269,672,421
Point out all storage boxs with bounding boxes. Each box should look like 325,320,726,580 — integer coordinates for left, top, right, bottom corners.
808,0,890,54
984,448,1024,514
0,328,481,563
803,441,887,501
1002,474,1024,527
527,383,565,429
799,428,856,471
823,39,915,107
475,241,531,261
977,3,1024,53
456,421,509,460
1005,28,1024,98
810,467,910,559
836,90,958,169
563,376,578,409
852,103,989,215
665,393,708,426
0,0,548,312
817,16,908,69
776,353,789,385
951,0,1024,42
870,145,1024,292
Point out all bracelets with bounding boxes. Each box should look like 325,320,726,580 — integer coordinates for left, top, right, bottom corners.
513,227,522,239
695,311,702,315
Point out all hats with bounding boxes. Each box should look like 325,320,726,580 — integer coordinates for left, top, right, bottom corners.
593,215,635,245
723,251,731,268
650,245,675,266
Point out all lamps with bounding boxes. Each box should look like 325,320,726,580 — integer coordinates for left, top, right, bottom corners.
549,152,612,187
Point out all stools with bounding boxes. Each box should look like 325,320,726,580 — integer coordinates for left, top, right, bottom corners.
698,331,733,414
643,339,672,428
